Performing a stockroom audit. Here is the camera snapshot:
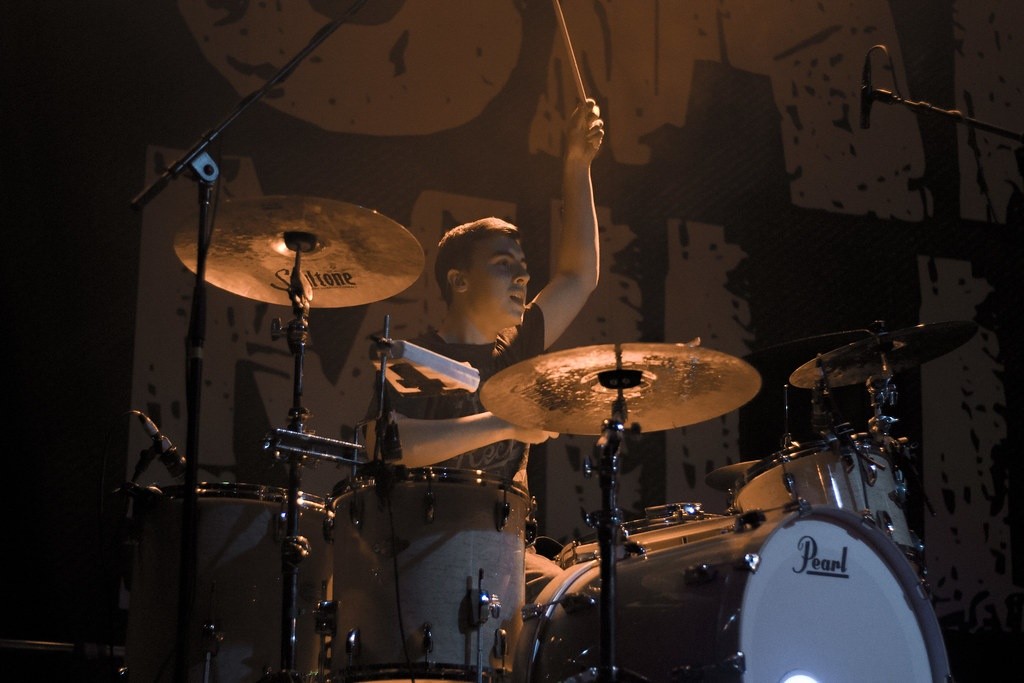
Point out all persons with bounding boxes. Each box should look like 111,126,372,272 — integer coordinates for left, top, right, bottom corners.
362,99,606,608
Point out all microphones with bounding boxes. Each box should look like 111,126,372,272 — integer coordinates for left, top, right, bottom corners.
859,53,874,129
810,354,834,434
138,412,186,478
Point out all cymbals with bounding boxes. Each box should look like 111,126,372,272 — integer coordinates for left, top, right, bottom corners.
479,340,764,438
704,458,762,493
788,315,981,391
172,194,427,311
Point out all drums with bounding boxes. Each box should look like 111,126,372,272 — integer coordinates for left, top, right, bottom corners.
323,464,533,683
506,503,953,683
727,433,928,584
114,477,331,683
